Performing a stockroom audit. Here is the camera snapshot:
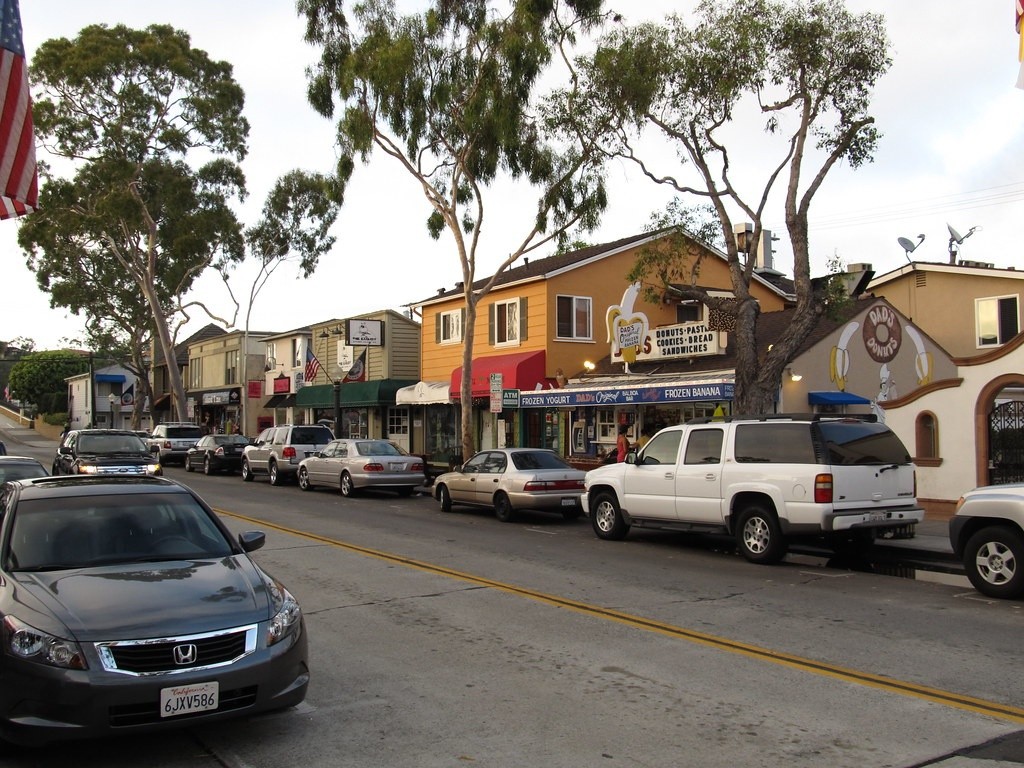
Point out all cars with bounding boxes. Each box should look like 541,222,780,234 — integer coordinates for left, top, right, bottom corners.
296,438,426,498
0,455,50,484
0,472,312,748
184,434,252,476
431,447,588,523
128,429,150,445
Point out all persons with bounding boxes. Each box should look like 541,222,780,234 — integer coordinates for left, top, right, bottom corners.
618,425,637,462
634,429,651,450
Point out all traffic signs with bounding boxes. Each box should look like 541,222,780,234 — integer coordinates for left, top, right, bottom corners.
489,372,503,414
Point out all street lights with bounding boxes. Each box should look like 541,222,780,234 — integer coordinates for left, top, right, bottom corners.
108,392,117,429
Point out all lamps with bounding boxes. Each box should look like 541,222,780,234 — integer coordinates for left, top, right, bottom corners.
788,367,802,381
264,357,283,372
584,360,596,374
319,322,345,337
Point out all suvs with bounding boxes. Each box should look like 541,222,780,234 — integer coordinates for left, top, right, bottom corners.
581,411,925,566
949,483,1024,602
145,420,206,467
51,427,165,478
239,423,337,486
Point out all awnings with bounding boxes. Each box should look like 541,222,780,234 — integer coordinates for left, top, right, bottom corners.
808,392,870,404
185,388,241,406
517,375,740,407
394,382,456,405
264,394,296,408
147,395,170,410
294,381,394,407
450,350,546,398
95,374,126,382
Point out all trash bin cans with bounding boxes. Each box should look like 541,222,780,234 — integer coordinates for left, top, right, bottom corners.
449,455,462,472
30,421,34,429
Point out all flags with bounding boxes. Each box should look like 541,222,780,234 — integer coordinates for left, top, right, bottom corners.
343,349,366,382
305,349,320,382
0,0,39,219
